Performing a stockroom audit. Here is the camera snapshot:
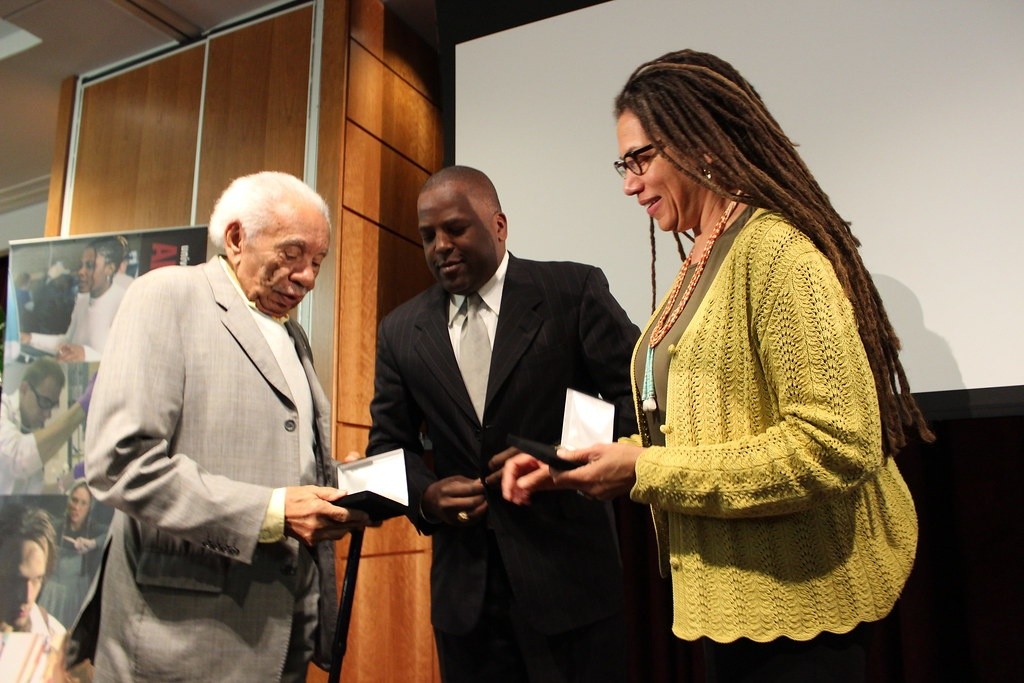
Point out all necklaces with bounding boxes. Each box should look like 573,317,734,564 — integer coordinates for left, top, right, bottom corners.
641,189,743,411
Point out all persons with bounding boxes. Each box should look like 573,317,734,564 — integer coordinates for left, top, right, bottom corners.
60,171,363,683
14,235,135,363
0,359,110,683
366,166,641,683
550,49,935,683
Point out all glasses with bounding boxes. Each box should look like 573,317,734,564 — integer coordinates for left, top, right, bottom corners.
614,140,662,179
27,384,59,410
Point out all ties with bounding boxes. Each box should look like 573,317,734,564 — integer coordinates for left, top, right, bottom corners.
459,293,491,426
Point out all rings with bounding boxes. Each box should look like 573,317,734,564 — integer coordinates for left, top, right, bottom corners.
458,511,468,522
